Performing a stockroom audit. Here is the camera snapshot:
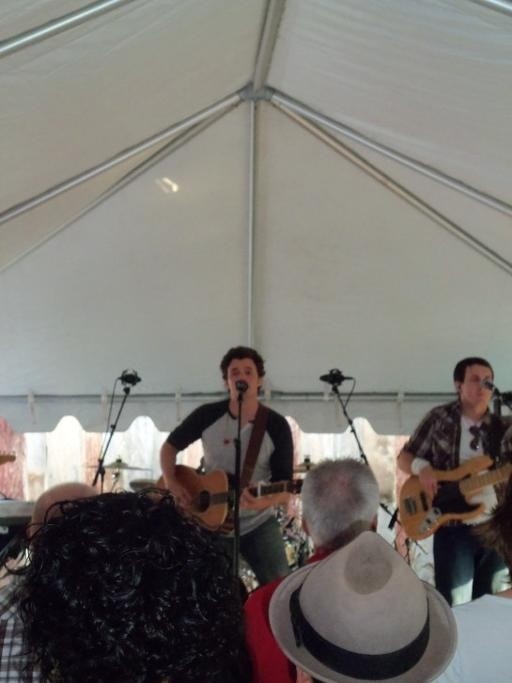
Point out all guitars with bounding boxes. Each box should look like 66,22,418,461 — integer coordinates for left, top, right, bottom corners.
153,466,302,536
399,457,511,540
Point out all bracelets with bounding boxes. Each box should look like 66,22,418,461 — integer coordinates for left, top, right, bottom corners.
411,457,430,476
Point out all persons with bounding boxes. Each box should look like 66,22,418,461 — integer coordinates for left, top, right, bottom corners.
397,357,512,607
160,346,293,584
0,482,98,683
0,488,257,683
243,457,379,683
432,472,512,683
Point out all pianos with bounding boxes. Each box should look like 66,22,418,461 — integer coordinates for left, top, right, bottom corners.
0,498,37,523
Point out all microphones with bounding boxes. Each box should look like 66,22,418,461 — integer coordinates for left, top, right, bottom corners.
236,380,249,392
320,374,353,382
121,368,138,387
482,379,501,397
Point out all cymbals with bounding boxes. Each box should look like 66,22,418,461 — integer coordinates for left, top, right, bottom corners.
94,463,153,473
130,479,164,497
